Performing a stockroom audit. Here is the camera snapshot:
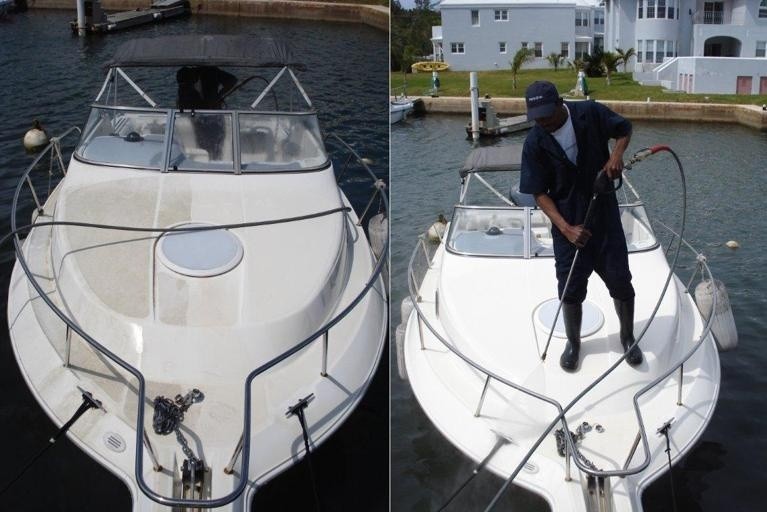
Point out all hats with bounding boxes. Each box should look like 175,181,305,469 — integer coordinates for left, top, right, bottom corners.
526,81,559,122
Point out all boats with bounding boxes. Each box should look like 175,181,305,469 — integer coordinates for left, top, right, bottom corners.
411,61,449,72
7,34,389,512
390,93,415,125
395,144,739,512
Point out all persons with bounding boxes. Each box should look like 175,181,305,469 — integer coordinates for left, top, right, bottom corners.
575,68,588,94
177,63,237,160
519,81,642,368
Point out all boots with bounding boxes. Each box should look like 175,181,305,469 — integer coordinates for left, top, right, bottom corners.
613,297,643,364
560,302,582,369
